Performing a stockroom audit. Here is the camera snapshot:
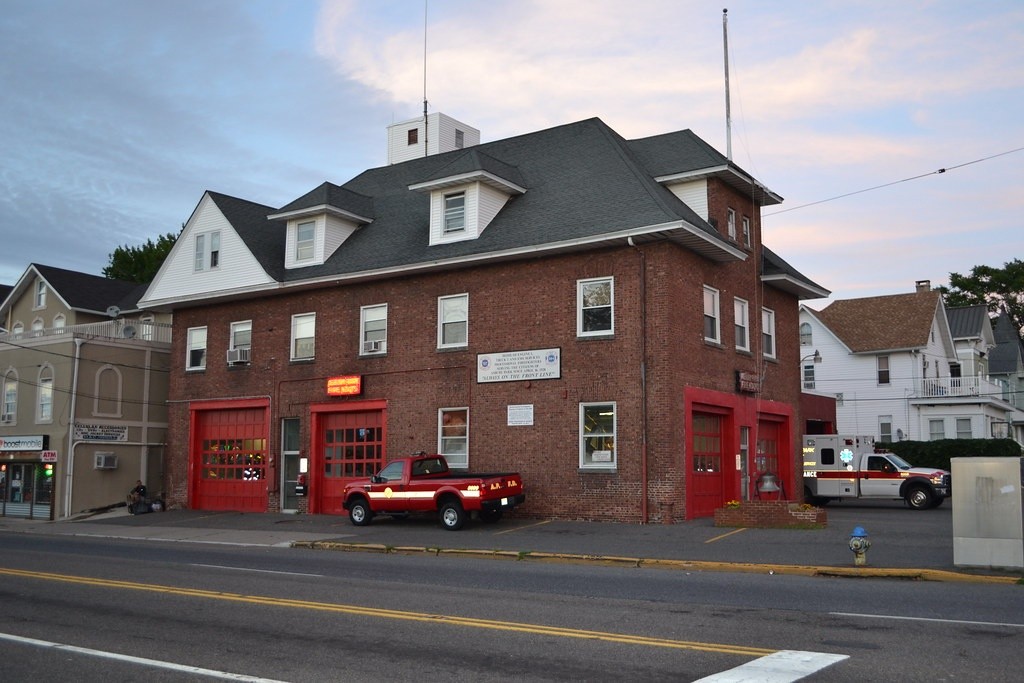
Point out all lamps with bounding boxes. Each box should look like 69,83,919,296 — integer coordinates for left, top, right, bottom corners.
799,349,822,367
123,326,137,338
107,304,121,321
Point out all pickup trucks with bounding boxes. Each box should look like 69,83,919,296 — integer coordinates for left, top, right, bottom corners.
341,450,526,531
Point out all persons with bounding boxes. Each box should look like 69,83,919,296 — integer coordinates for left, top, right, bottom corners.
243,453,261,481
130,480,146,497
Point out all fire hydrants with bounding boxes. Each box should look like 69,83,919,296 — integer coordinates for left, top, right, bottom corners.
849,523,871,565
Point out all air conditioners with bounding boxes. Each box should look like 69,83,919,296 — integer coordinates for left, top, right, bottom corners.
94,451,118,469
226,348,250,365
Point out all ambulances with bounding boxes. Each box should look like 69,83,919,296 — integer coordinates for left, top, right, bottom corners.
802,434,952,510
